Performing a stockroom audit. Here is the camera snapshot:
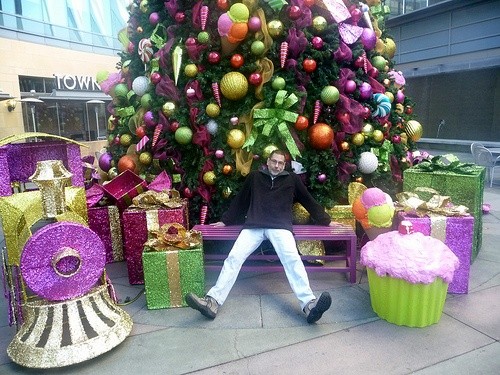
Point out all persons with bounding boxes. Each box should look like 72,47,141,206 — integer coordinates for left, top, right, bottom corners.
183,148,343,324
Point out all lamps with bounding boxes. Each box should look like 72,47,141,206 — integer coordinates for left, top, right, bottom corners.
6,99,16,112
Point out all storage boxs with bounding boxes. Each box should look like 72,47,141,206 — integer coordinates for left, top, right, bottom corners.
397,211,475,294
402,165,487,265
89,168,207,310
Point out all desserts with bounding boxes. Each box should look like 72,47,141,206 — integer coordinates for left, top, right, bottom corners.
360,221,460,327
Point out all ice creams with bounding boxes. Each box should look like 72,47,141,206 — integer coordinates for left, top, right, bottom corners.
172,46,182,86
218,3,249,54
352,187,395,241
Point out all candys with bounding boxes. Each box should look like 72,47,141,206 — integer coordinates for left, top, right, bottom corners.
137,38,153,73
372,93,391,118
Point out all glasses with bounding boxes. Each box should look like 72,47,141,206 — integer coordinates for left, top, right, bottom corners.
270,159,285,167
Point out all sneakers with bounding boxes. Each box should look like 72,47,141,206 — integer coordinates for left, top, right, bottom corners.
305,291,332,322
185,293,220,319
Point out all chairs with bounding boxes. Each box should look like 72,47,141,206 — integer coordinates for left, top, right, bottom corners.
470,142,500,188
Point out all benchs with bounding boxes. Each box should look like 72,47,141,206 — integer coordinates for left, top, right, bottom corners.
190,224,357,285
